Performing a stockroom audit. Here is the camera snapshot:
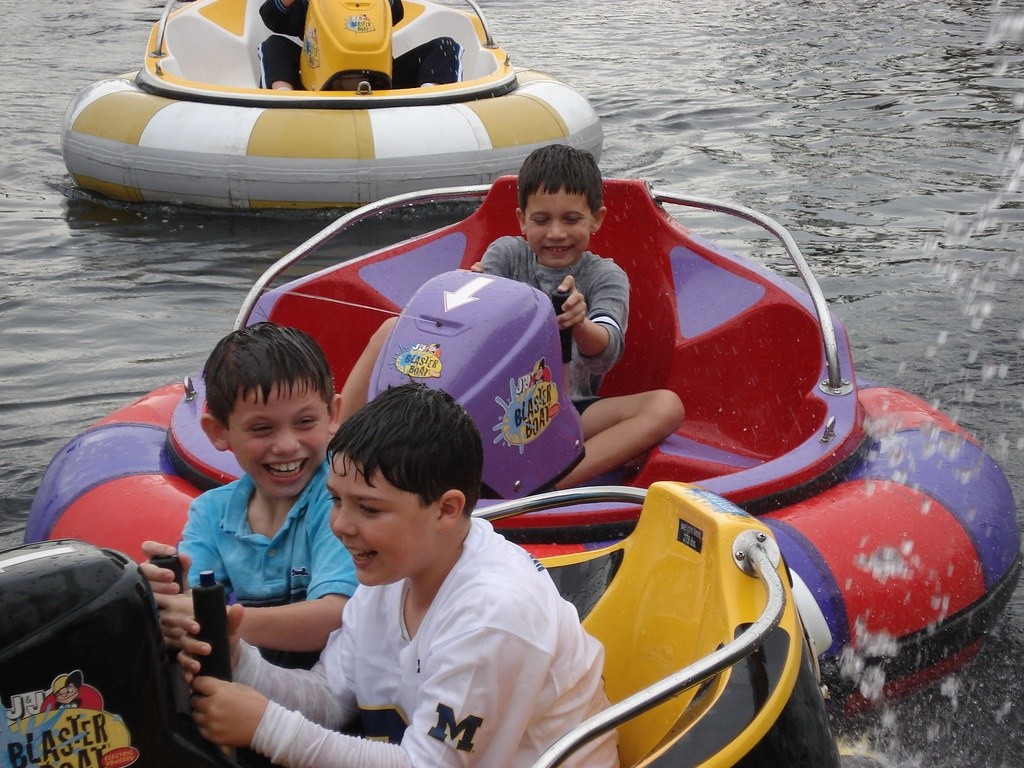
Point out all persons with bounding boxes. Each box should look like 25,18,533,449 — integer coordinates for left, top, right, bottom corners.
339,142,685,490
257,0,461,91
140,321,358,649
177,380,619,768
